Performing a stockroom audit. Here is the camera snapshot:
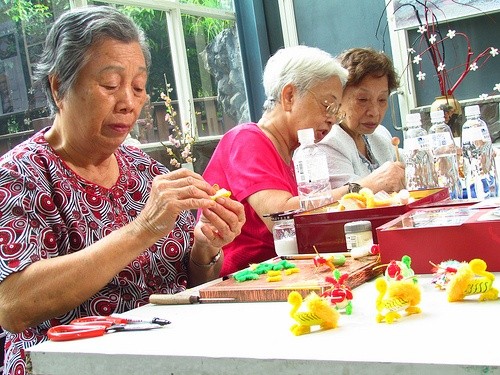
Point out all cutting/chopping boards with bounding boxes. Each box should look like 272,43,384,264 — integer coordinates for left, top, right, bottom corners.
198,254,386,302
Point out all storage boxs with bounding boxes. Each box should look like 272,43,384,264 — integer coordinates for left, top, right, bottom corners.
293,188,500,273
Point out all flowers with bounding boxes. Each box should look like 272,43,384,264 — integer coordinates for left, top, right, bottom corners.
146,72,201,169
376,1,498,100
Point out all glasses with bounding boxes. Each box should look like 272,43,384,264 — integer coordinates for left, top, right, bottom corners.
294,82,346,125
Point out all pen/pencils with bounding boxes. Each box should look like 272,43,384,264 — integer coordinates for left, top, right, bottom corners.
392,137,400,160
280,256,315,260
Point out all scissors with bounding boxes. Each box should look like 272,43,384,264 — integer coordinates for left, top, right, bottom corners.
47,315,164,341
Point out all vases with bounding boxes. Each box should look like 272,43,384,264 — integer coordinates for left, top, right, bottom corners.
430,96,465,138
180,162,194,173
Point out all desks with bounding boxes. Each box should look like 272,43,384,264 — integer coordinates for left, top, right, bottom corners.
29,252,500,375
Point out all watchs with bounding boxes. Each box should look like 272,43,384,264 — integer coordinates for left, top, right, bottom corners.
191,244,221,269
343,182,362,193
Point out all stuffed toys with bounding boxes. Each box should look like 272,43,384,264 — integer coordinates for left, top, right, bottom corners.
289,253,498,334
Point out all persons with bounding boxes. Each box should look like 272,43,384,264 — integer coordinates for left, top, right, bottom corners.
196,45,407,276
0,6,245,375
316,48,406,189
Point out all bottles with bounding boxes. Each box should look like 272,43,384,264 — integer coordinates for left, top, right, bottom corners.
458,105,498,201
427,110,459,201
293,127,333,211
272,220,299,256
402,112,438,190
343,220,374,254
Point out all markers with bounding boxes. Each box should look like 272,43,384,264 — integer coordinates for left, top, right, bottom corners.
111,313,171,325
